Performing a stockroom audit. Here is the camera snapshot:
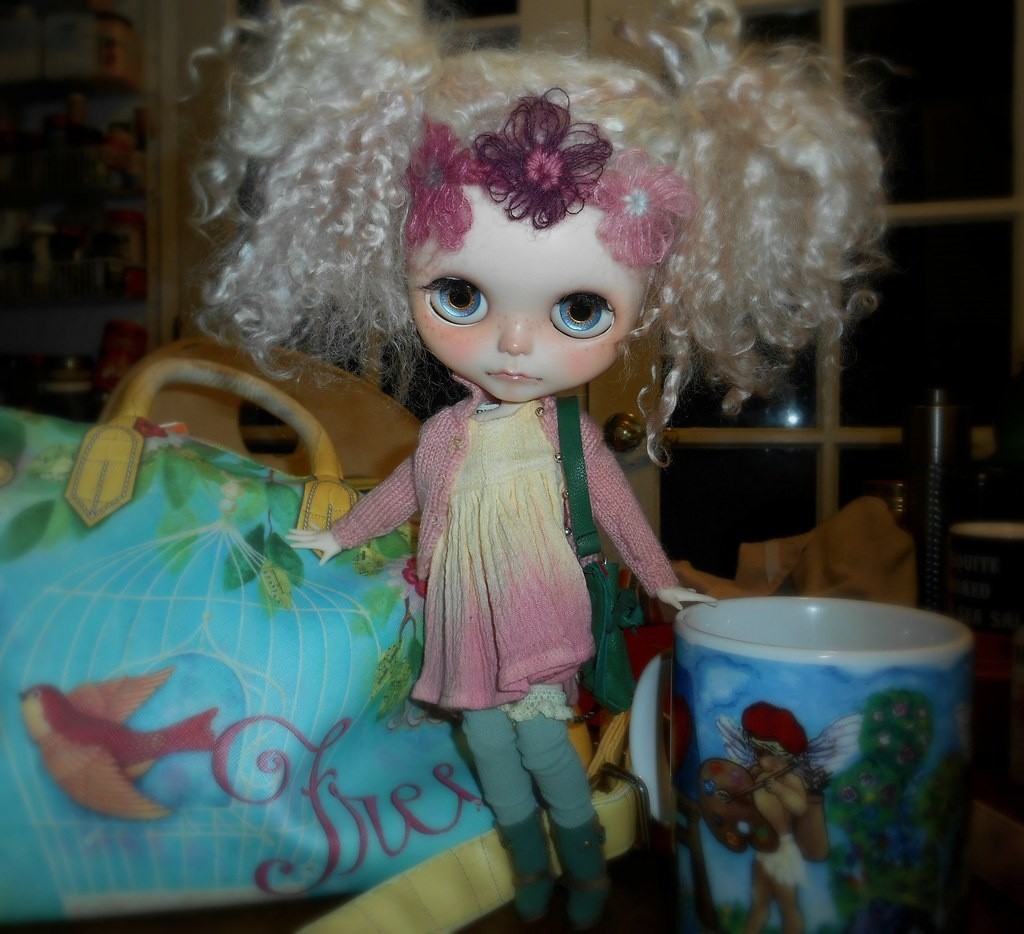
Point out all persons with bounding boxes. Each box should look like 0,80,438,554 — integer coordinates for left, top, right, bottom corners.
188,1,888,934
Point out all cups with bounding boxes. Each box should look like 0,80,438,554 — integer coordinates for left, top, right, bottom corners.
948,521,1024,635
630,597,979,934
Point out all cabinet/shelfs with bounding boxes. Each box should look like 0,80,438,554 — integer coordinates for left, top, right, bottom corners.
0,0,184,357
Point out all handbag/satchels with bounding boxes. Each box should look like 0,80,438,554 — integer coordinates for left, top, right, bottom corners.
0,359,653,934
